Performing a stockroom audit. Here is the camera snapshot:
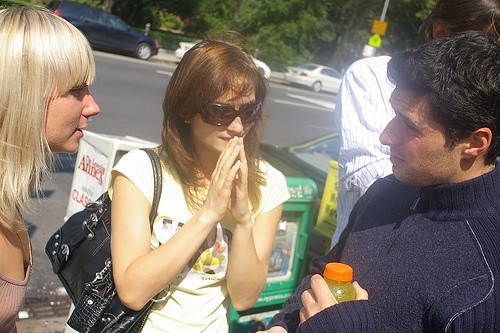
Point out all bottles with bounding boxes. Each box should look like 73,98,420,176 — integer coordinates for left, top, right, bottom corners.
322,262,356,303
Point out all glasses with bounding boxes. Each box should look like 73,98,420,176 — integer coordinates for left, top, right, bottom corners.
200,102,258,126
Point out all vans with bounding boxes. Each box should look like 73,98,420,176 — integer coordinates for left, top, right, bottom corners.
44,0,160,60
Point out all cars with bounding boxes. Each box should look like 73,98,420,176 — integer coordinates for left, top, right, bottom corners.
257,132,342,231
284,63,343,93
174,39,271,81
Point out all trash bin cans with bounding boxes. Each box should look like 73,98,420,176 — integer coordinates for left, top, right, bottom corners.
228,146,318,323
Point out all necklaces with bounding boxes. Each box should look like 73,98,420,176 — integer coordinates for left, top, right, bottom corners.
16,232,33,267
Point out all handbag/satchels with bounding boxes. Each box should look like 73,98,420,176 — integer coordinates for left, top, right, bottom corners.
44,148,164,333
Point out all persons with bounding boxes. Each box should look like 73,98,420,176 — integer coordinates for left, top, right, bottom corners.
255,30,500,333
328,0,500,252
108,39,291,333
0,3,100,333
